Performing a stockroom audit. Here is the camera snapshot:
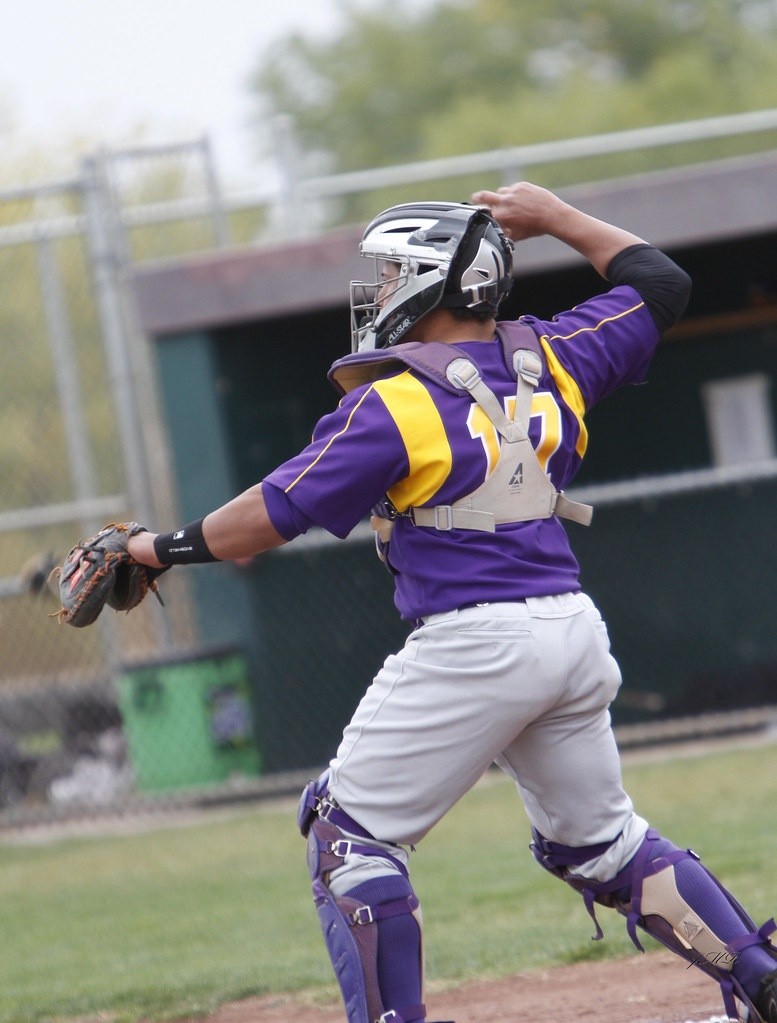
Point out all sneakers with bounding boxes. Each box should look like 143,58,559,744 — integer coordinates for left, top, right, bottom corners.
744,969,777,1023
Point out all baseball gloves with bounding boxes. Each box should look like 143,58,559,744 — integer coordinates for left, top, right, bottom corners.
59,519,162,628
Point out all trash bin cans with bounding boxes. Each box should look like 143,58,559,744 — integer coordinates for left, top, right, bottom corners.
113,643,262,793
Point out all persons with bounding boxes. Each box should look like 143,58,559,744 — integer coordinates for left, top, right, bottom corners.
58,181,777,1023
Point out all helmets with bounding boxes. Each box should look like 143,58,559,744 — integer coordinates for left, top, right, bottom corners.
352,202,515,352
